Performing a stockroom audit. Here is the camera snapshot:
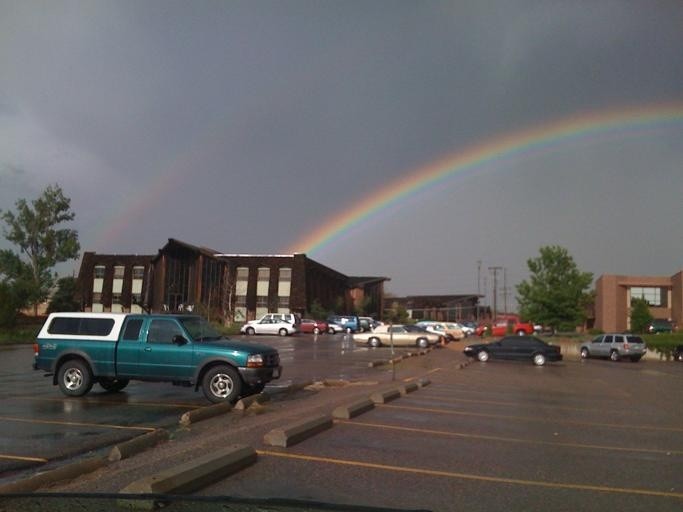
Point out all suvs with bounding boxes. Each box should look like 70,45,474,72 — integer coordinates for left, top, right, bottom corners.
576,332,648,362
643,318,676,334
245,312,301,325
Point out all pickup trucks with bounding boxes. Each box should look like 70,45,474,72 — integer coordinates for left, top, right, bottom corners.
31,308,284,410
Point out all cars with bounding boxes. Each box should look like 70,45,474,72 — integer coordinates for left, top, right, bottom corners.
240,318,296,336
462,334,563,366
351,324,443,349
297,308,547,339
